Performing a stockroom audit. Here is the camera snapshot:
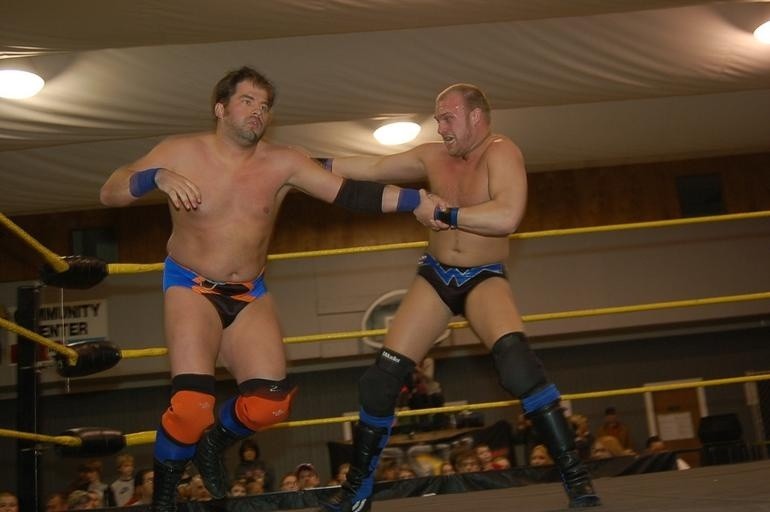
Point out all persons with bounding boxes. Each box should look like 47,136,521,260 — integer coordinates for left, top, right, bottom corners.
1,400,690,511
99,65,447,512
407,354,449,437
314,83,600,512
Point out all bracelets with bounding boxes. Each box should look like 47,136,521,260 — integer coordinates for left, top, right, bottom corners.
311,157,334,173
396,187,432,214
434,205,458,229
129,168,159,198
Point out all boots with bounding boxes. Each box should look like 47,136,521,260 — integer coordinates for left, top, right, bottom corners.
317,406,395,512
191,394,256,500
521,384,601,509
152,422,200,511
